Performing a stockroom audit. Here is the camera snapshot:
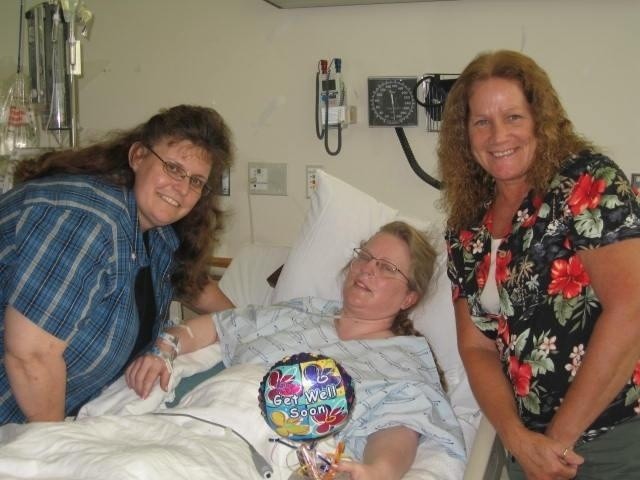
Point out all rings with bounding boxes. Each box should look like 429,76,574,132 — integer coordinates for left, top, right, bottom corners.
563,448,568,457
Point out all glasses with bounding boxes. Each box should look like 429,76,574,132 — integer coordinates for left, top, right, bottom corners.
352,247,414,285
148,146,213,196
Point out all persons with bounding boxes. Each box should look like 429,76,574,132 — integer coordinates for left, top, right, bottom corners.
0,105,235,426
431,49,640,480
124,221,467,479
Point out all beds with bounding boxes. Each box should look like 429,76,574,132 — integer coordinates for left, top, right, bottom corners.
1,238,506,480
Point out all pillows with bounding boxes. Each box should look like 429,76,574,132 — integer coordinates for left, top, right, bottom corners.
273,166,467,373
